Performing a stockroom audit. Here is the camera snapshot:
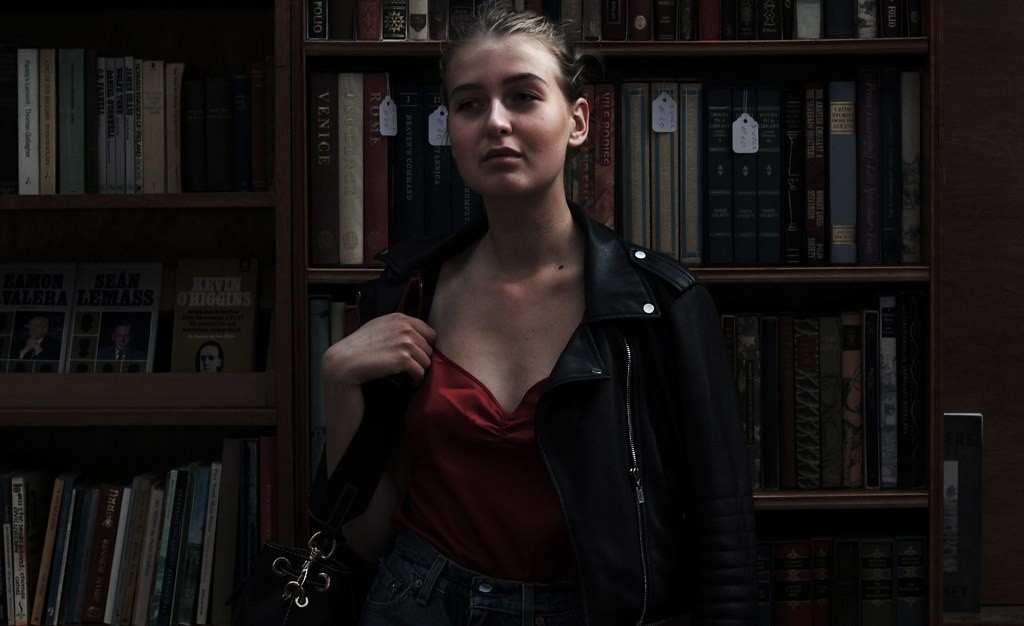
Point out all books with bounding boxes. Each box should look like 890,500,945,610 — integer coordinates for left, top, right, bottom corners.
719,294,927,492
310,69,483,266
0,259,256,373
307,0,925,40
0,434,277,626
563,71,926,267
0,43,272,192
309,293,362,484
756,534,929,626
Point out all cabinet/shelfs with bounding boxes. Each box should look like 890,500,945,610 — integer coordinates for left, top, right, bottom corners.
293,0,935,626
0,0,291,626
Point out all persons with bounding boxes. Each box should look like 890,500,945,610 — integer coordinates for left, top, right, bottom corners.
12,315,54,359
97,320,147,360
306,6,762,626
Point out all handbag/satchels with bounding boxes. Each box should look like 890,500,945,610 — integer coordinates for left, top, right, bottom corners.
231,541,362,626
